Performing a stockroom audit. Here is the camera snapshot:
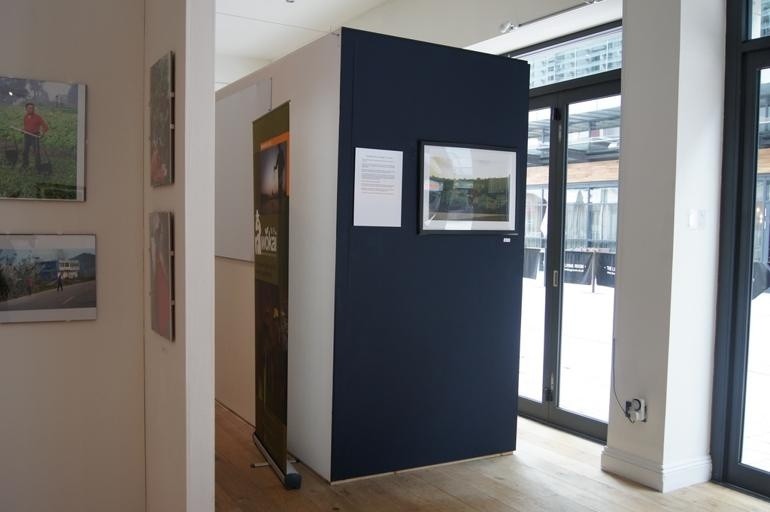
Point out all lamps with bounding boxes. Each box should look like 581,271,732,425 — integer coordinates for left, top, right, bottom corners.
498,20,516,34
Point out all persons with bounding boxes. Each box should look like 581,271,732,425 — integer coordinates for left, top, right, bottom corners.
272,144,285,194
20,102,49,175
25,276,33,295
56,274,64,291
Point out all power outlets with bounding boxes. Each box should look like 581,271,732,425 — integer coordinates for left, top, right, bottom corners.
626,400,646,422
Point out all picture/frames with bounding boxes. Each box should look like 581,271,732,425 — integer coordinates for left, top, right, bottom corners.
418,140,521,236
